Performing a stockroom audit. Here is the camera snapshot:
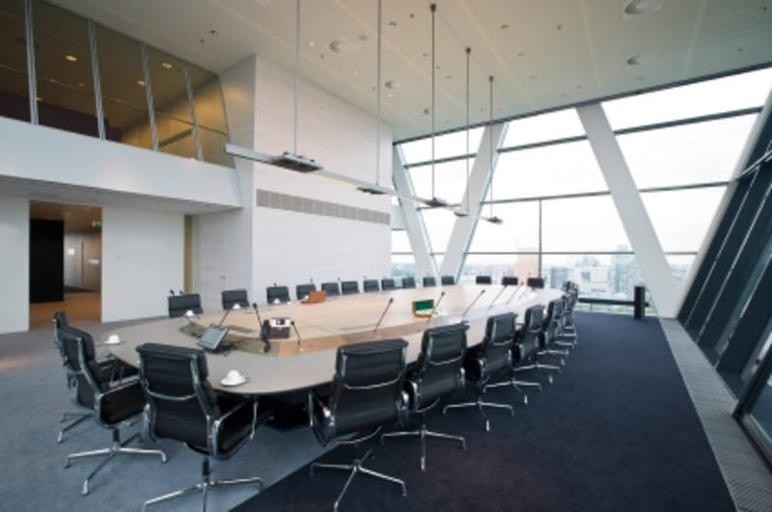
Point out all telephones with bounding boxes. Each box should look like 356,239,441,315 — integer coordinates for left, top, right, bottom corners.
259,316,293,339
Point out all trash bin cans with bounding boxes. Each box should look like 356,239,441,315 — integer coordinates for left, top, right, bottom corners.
634,286,646,321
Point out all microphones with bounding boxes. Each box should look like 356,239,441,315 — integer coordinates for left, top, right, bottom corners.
178,289,197,315
488,283,509,310
253,302,264,328
371,297,393,336
218,302,235,327
272,272,449,293
506,278,541,304
462,290,486,320
290,320,301,347
425,291,445,329
171,289,177,319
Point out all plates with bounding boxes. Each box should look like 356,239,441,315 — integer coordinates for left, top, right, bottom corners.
221,377,246,386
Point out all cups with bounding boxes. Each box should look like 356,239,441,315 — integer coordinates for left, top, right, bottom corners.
104,340,122,345
225,369,240,382
109,335,120,342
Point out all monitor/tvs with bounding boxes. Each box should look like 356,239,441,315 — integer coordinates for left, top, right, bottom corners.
197,322,229,352
